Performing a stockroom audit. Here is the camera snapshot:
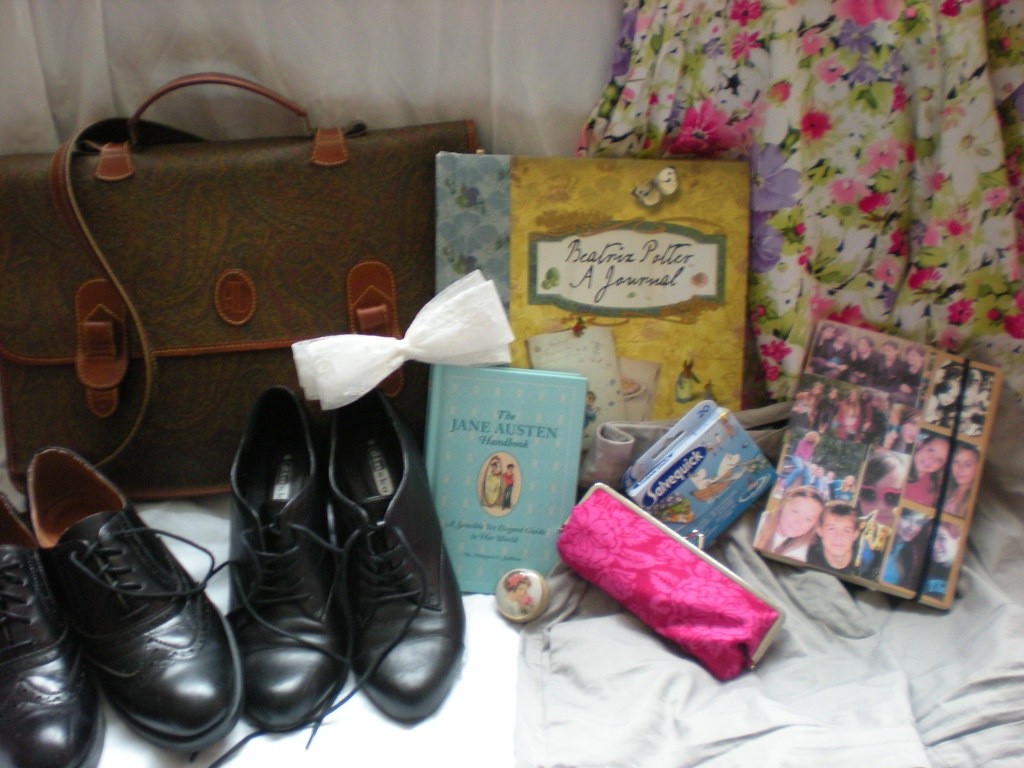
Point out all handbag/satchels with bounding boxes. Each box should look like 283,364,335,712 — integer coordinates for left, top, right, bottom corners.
0,72,477,500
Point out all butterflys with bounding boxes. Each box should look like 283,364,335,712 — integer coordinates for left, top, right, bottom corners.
632,165,681,208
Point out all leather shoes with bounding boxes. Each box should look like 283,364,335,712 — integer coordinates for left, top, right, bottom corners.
203,385,353,734
327,384,464,727
27,446,244,754
0,491,99,768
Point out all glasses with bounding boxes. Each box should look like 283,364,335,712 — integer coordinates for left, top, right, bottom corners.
859,484,904,508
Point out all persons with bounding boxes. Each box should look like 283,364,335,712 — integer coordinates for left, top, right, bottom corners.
861,452,908,528
754,320,995,604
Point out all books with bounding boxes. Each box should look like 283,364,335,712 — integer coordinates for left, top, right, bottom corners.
423,367,589,597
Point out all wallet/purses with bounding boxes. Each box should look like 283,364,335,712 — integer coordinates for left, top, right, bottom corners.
555,482,785,683
